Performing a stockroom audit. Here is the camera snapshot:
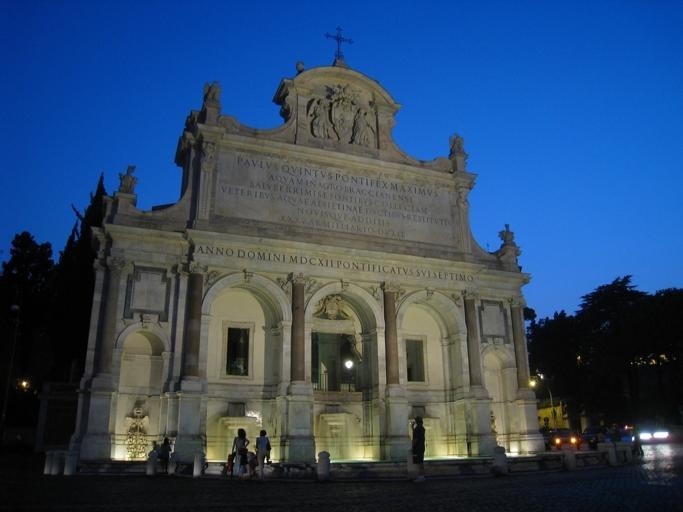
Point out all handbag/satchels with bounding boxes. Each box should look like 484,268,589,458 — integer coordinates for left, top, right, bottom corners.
238,447,249,454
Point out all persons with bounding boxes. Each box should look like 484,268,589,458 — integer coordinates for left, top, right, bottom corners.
411,415,425,483
148,438,172,474
255,430,272,479
232,428,250,480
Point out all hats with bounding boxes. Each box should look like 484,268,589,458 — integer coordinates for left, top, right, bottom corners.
414,416,425,425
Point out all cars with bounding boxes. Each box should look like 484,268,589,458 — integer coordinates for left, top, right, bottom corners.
579,423,638,455
540,427,581,452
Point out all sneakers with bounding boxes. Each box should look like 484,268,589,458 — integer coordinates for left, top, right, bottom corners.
414,477,427,483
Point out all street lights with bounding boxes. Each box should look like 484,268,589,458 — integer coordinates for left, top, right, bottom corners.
529,379,555,436
